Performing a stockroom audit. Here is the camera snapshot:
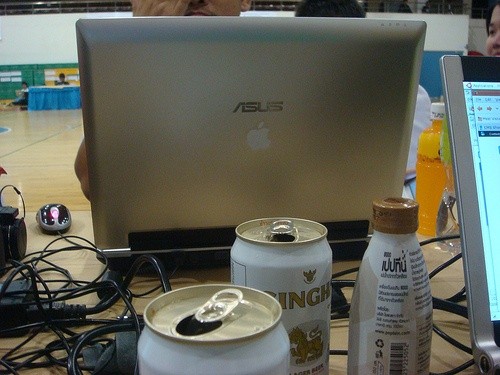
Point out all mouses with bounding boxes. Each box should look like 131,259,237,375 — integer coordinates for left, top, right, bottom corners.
35,203,71,234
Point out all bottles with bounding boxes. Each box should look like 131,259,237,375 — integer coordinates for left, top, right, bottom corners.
348,197,433,375
417,103,463,254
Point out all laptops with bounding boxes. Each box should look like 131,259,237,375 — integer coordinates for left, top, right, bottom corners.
71,16,428,270
440,54,500,375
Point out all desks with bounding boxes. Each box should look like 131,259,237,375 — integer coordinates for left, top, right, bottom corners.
0,211,475,375
28,85,81,111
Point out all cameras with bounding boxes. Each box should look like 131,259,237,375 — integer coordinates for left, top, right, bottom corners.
0,206,27,266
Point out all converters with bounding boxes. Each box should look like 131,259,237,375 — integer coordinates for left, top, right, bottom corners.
0,277,38,338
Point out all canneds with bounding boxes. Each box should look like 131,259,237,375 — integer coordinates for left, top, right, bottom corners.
230,216,333,375
137,284,292,375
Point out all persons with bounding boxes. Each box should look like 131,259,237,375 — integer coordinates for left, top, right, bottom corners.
74,0,251,201
485,0,500,57
5,81,29,106
295,0,433,176
55,73,69,85
